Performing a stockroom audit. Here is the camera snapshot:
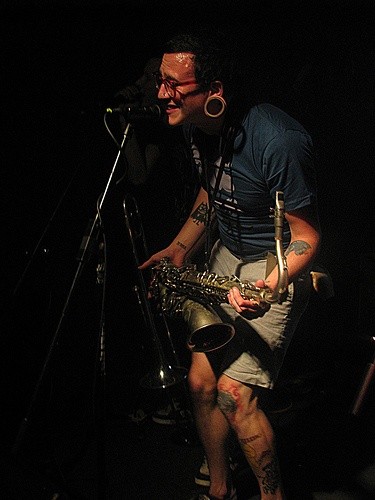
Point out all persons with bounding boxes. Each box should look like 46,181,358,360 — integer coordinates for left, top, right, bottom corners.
116,46,293,424
137,30,323,500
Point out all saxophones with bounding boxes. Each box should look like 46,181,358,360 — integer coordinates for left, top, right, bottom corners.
148,191,288,353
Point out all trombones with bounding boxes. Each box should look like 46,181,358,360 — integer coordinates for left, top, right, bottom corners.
120,195,193,425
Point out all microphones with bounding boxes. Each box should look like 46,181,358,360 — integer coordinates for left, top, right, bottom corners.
120,103,165,120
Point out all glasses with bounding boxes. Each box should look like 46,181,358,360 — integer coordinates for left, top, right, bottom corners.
157,70,206,98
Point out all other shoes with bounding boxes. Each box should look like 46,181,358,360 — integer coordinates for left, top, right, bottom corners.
192,481,237,500
194,456,238,486
258,395,292,413
152,398,184,425
